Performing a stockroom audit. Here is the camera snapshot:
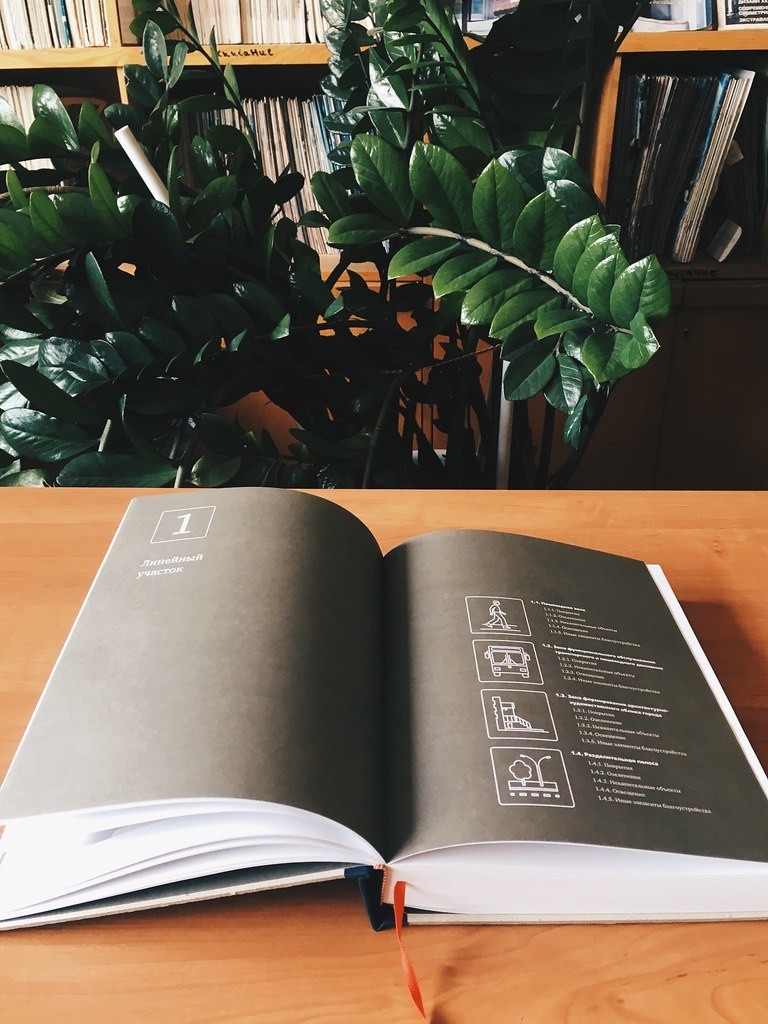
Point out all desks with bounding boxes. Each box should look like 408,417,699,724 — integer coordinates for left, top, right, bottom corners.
0,484,768,1024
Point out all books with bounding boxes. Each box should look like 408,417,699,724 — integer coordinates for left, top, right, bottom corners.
0,1,768,315
0,488,768,931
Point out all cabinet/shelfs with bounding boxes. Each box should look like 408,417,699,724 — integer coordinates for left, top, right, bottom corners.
0,0,767,488
